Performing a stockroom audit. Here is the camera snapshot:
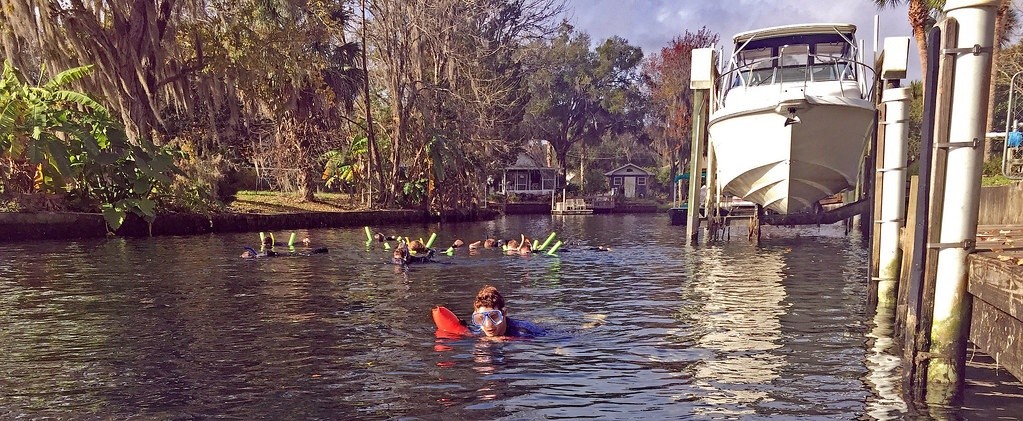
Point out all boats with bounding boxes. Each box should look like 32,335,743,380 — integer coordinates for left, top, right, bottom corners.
707,22,884,218
549,188,593,215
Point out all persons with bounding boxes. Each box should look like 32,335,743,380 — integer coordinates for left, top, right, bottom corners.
468,287,554,337
240,232,613,266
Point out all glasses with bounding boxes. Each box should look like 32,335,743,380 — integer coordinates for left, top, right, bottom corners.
472,310,504,326
